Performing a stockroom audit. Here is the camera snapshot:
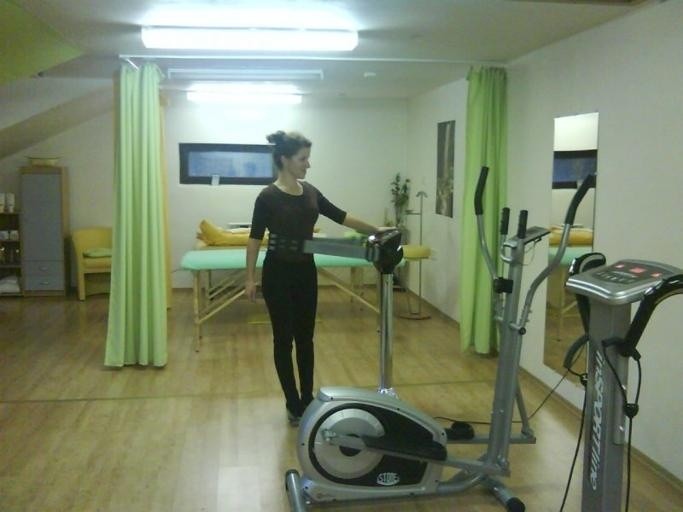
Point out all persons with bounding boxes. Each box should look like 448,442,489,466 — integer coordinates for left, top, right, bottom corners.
243,128,397,426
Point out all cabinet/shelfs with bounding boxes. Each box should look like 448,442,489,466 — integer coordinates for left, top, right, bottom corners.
0,167,69,298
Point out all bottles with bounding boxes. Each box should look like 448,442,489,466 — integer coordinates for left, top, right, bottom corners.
0,230,19,266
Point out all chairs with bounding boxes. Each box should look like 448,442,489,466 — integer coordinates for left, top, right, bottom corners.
68,225,114,302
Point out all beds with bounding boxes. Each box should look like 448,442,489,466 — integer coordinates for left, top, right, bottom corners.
179,228,408,354
547,224,591,344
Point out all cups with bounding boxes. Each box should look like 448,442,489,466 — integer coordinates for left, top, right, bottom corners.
0,192,15,213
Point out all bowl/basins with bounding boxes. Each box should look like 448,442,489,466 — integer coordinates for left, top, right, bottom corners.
21,155,61,167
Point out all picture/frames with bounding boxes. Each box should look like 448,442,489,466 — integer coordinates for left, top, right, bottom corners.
176,142,277,186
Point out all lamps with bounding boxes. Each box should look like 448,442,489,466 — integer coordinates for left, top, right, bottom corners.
140,25,361,54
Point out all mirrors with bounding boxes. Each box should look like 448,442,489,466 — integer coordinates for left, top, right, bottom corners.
541,112,602,389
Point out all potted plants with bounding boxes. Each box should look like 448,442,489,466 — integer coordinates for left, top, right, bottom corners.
381,173,410,284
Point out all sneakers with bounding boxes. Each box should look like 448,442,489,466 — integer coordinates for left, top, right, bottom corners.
285,410,301,427
